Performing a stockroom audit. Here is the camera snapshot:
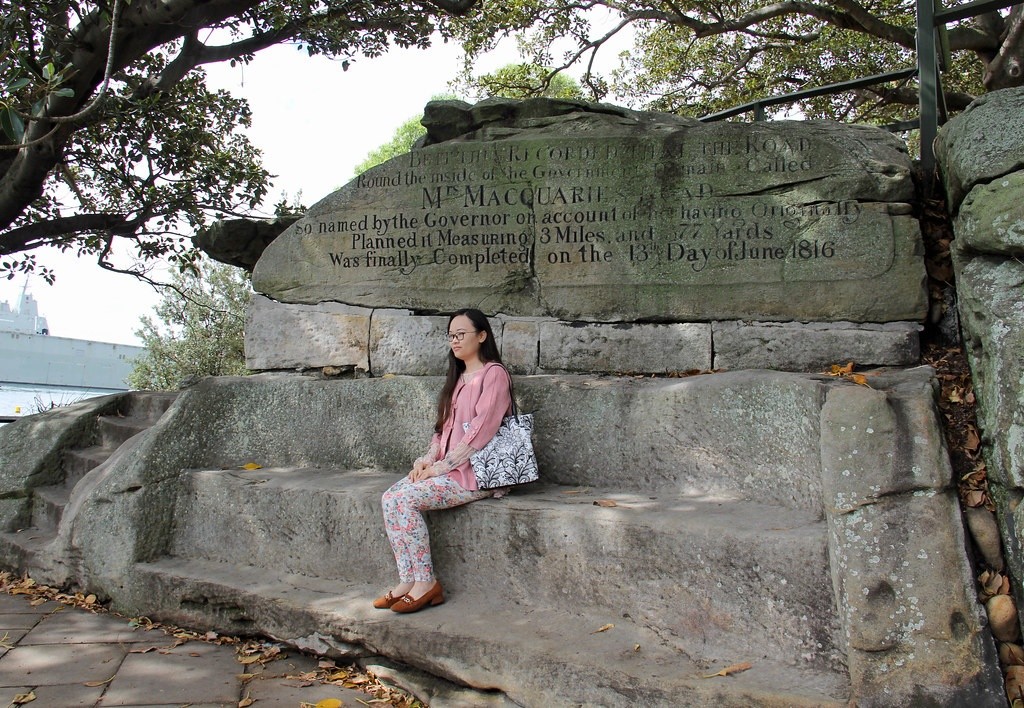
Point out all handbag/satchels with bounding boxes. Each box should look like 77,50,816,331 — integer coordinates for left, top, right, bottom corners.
463,365,538,489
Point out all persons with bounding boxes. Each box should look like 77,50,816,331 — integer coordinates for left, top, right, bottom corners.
373,308,515,613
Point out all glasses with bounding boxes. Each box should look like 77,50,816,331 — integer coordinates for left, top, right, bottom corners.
445,331,479,342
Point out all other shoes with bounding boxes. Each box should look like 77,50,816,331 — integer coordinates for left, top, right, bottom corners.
373,591,410,609
391,580,444,612
